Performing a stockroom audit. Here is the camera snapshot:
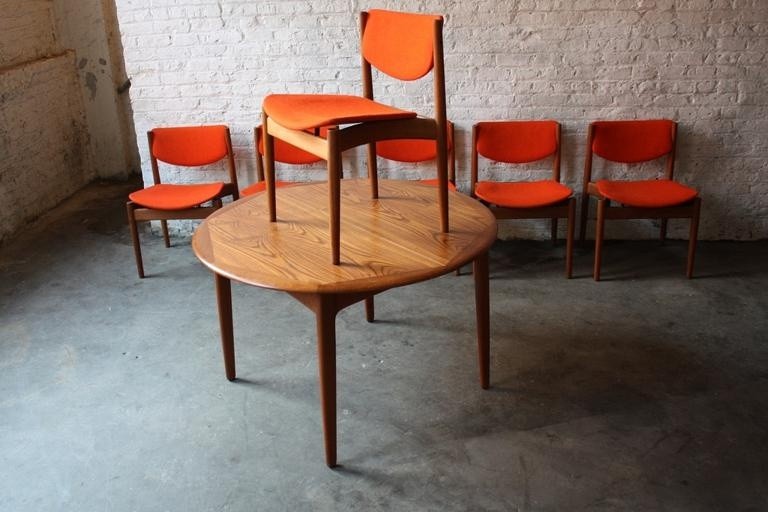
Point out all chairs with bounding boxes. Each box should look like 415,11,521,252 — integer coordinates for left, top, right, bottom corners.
259,6,453,267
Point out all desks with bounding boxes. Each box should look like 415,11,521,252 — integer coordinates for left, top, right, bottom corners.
190,176,499,470
